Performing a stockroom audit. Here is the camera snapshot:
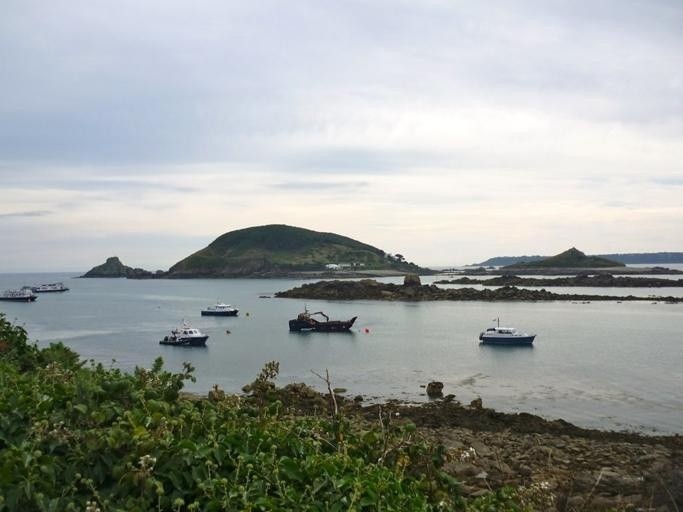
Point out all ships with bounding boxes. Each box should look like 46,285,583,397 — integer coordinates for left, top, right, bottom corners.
0,287,37,302
34,281,69,292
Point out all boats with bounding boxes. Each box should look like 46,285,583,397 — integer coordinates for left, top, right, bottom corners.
159,320,209,347
480,318,536,346
201,298,239,317
287,311,358,332
